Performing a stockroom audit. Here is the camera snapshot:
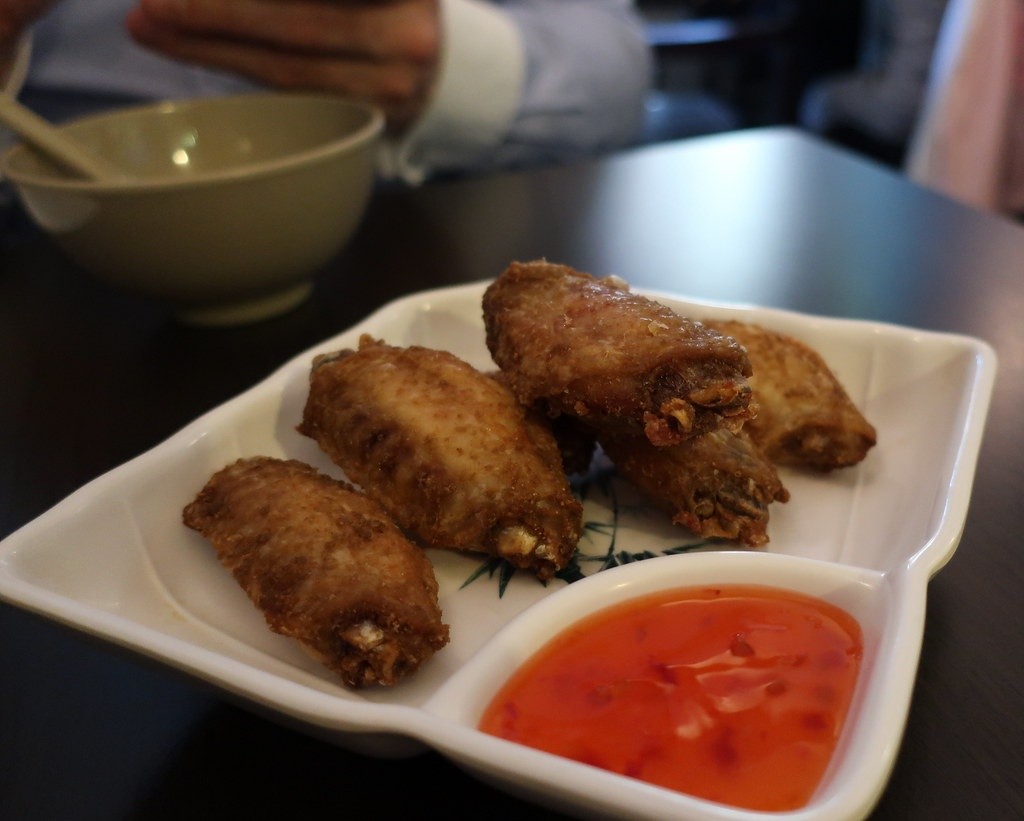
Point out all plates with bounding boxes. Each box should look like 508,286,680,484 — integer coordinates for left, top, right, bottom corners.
0,277,998,821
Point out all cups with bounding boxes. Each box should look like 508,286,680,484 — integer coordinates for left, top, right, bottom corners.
389,0,523,184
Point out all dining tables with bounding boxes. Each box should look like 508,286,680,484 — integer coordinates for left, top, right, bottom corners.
0,121,1024,821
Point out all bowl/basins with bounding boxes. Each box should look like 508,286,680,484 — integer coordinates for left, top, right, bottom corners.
0,91,387,324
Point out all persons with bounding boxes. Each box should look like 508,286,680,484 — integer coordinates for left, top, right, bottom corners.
0,0,654,189
